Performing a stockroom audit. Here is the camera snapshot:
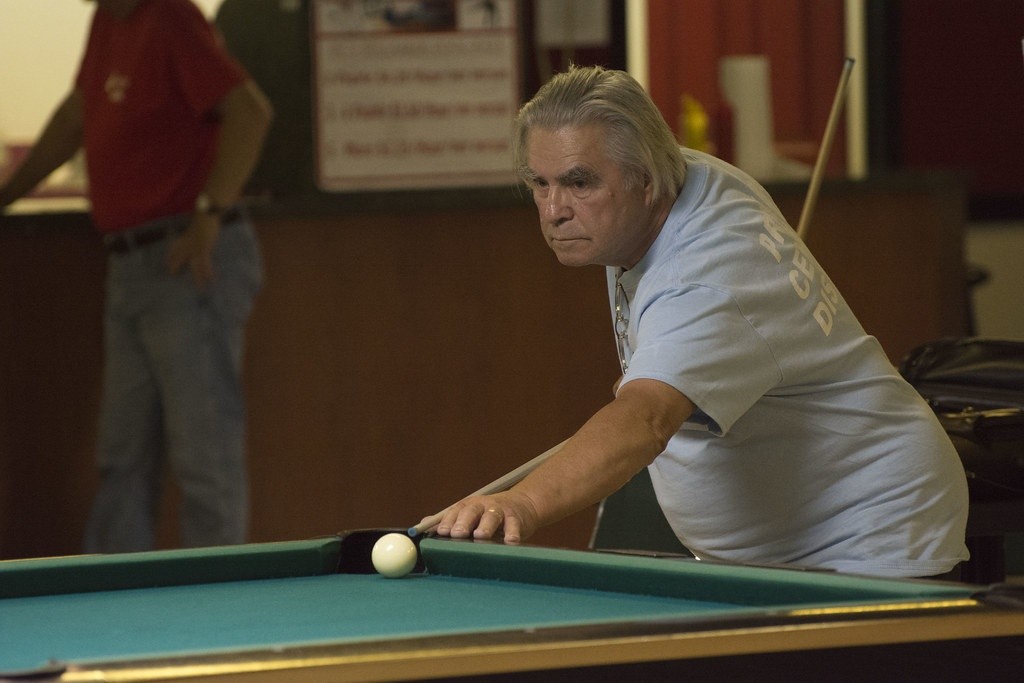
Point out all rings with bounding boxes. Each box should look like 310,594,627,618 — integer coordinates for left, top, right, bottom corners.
487,509,503,528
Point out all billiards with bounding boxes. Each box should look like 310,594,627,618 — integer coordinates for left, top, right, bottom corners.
371,532,417,579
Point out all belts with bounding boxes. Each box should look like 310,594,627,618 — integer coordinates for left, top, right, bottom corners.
108,211,239,255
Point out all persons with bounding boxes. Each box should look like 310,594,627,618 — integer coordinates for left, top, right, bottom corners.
421,69,971,578
0,0,270,551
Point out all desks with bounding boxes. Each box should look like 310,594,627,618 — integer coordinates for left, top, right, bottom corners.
0,527,1024,683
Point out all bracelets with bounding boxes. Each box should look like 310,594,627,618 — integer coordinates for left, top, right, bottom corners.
197,195,228,215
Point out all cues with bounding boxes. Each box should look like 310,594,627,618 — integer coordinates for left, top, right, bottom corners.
407,434,573,537
797,56,856,239
